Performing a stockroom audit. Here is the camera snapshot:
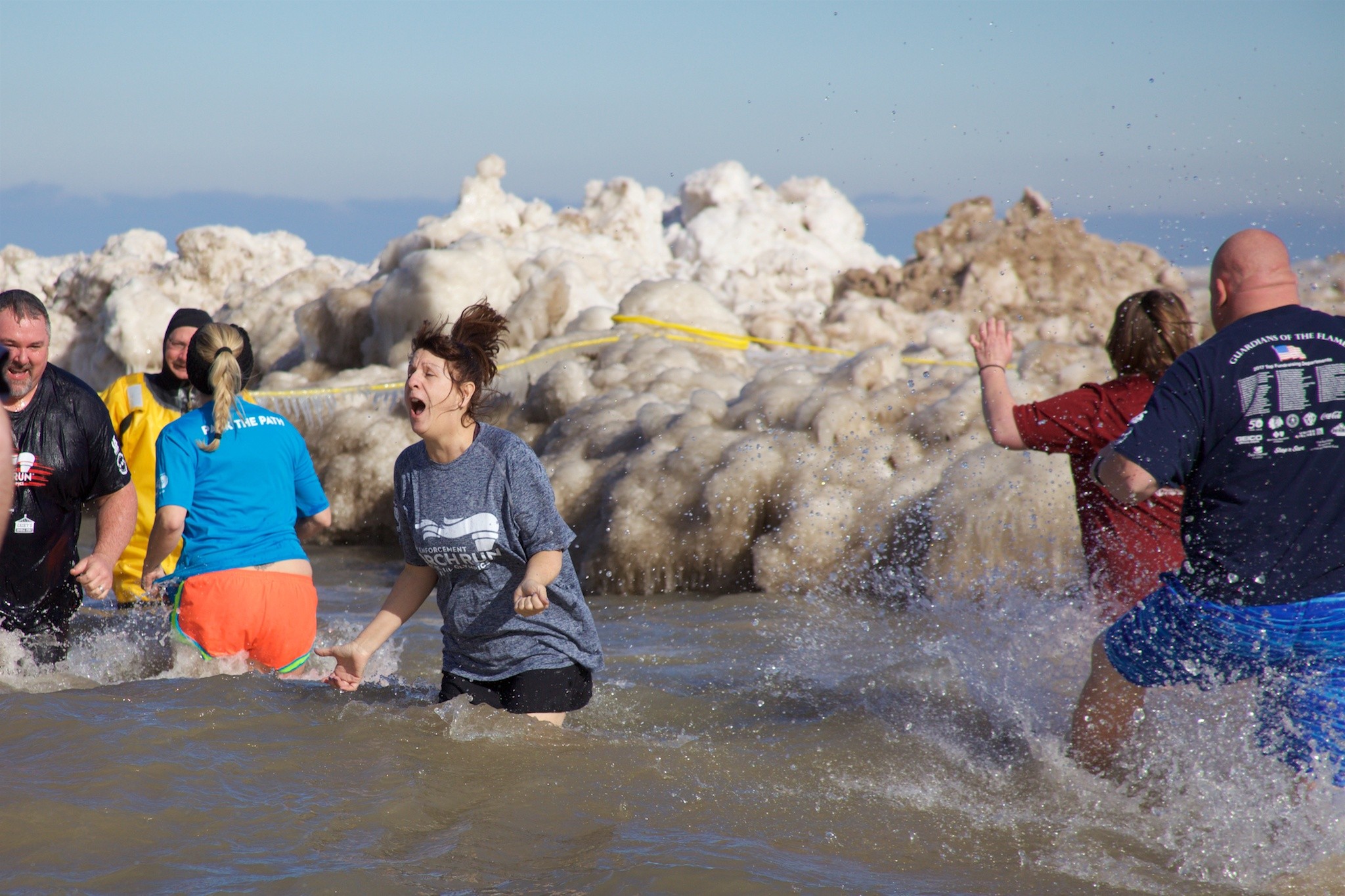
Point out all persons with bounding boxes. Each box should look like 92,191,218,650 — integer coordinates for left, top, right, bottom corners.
310,301,607,725
960,286,1201,629
0,289,137,672
0,344,16,541
1062,230,1344,803
138,319,337,684
95,307,258,614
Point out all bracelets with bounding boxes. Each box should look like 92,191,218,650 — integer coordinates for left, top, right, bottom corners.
977,363,1005,375
1088,456,1104,487
